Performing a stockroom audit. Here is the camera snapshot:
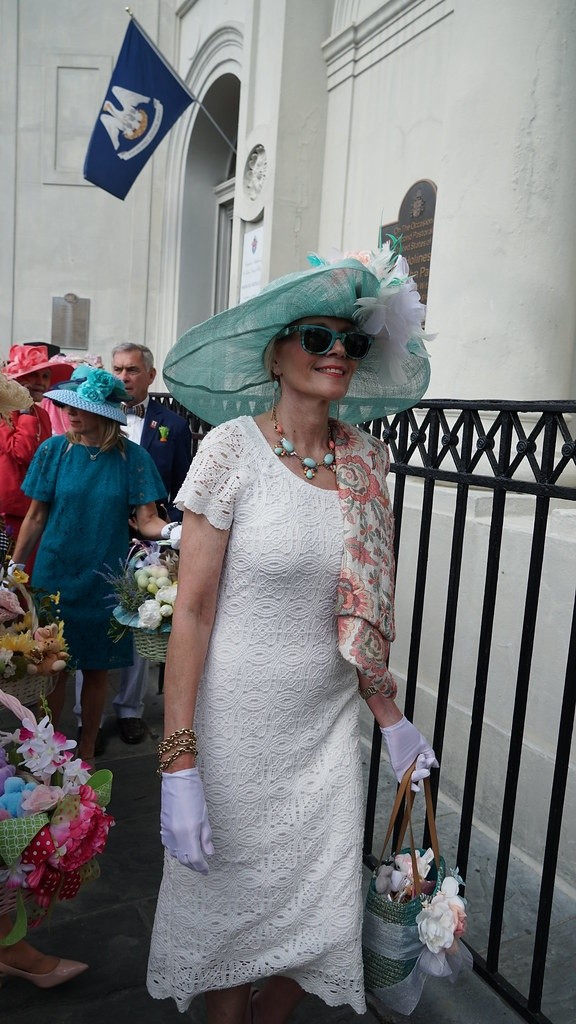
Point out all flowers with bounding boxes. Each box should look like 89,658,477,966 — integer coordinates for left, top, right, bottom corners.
0,345,47,373
395,847,467,952
0,556,80,687
75,368,118,404
0,692,115,874
93,538,184,644
344,233,441,393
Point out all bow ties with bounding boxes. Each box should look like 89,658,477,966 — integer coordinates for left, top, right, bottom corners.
121,403,145,418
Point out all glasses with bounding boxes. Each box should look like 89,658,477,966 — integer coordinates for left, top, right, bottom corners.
277,325,373,361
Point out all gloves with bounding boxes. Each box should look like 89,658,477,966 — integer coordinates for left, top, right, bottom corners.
379,713,439,793
161,522,183,549
161,767,215,875
2,559,25,591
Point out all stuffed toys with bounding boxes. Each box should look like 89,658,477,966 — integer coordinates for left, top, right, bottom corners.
0,745,100,908
26,625,70,675
0,589,26,631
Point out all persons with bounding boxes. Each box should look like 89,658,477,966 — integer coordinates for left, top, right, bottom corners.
146,243,440,1024
0,913,88,987
2,341,73,585
3,366,183,770
72,342,191,743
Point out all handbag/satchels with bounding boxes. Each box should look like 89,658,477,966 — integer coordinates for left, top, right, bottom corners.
362,846,468,1015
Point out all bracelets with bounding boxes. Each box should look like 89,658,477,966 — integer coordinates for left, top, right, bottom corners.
167,522,183,535
19,409,31,414
156,728,199,771
359,687,378,698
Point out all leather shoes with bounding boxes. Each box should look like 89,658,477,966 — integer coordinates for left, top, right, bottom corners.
77,727,102,755
119,717,142,744
0,957,89,989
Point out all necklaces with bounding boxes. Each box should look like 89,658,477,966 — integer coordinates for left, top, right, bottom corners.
83,436,109,459
271,407,338,477
29,405,41,442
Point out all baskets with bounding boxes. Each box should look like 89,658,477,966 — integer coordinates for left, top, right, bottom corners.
0,573,60,711
0,689,55,913
124,549,181,662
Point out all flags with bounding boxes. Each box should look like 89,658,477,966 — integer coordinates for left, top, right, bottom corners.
83,18,196,202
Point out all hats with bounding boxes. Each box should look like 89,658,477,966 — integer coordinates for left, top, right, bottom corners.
163,211,438,428
2,344,76,390
42,364,134,426
0,374,34,413
84,354,103,368
